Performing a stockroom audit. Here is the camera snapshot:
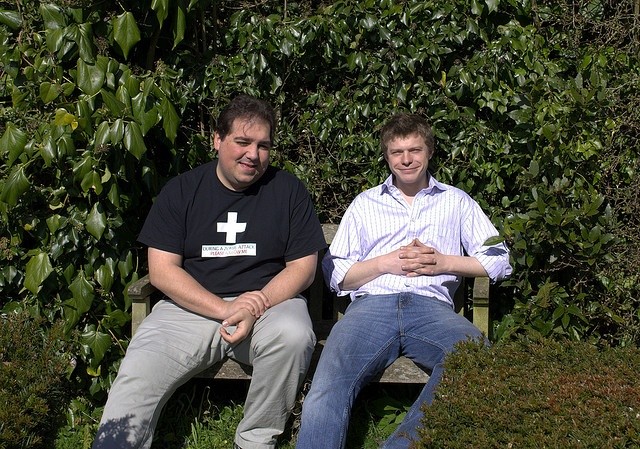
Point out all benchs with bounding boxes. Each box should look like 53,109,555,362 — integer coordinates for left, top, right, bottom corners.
127,224,489,384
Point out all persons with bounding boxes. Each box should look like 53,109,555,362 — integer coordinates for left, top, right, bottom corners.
296,113,516,449
97,96,322,448
